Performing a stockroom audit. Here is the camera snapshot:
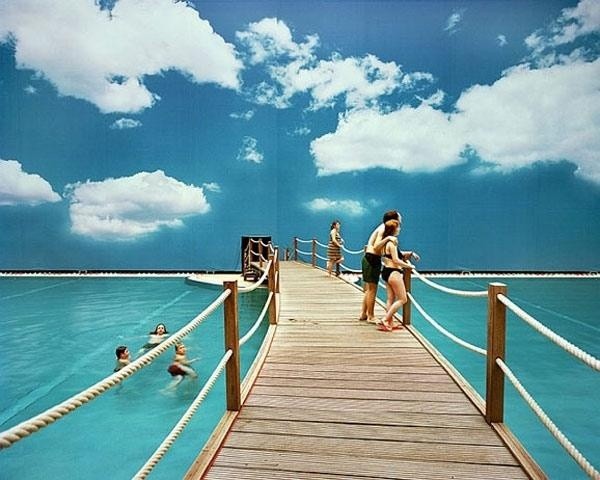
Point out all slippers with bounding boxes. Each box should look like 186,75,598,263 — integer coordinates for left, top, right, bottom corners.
361,315,403,330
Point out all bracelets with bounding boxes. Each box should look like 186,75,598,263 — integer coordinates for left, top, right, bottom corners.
411,251,413,256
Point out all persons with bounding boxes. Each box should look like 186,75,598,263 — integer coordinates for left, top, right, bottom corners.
375,219,421,332
161,341,200,397
136,323,170,359
113,346,132,372
360,210,402,325
327,219,345,279
